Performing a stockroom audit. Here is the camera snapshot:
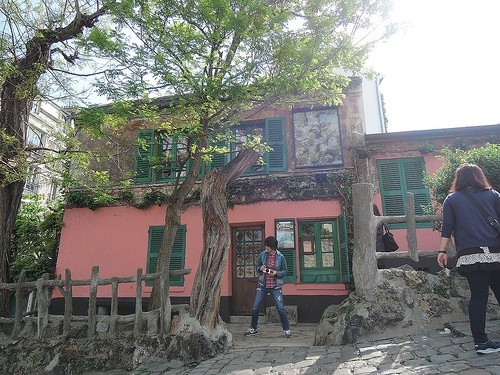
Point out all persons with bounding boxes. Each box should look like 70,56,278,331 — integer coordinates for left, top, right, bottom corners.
437,162,500,355
373,203,389,269
244,236,291,338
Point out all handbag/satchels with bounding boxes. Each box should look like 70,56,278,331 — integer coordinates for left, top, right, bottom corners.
382,231,399,251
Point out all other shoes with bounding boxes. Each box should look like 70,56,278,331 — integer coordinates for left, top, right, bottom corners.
244,327,259,336
283,329,292,339
474,339,500,354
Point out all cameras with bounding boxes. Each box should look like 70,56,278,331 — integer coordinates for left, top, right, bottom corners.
263,268,270,274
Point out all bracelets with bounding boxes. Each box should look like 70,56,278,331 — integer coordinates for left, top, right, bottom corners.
438,250,448,253
